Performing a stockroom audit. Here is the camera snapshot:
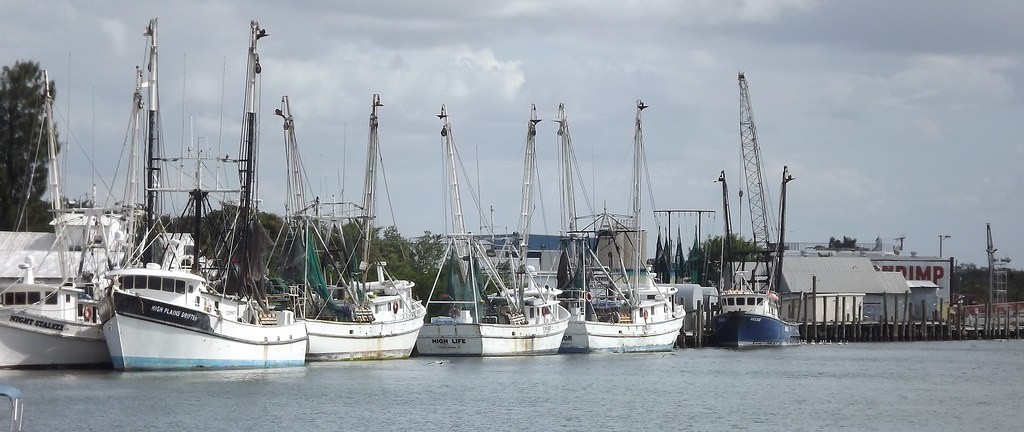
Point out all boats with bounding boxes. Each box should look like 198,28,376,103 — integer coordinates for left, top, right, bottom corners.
297,95,428,362
414,103,573,357
91,20,307,373
558,101,687,355
1,63,142,370
709,165,803,348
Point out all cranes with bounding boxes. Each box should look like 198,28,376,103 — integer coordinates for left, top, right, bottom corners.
736,72,773,253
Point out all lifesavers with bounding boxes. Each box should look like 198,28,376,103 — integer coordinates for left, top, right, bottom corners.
84,306,92,322
586,291,593,302
644,310,648,319
393,301,398,313
542,307,547,316
